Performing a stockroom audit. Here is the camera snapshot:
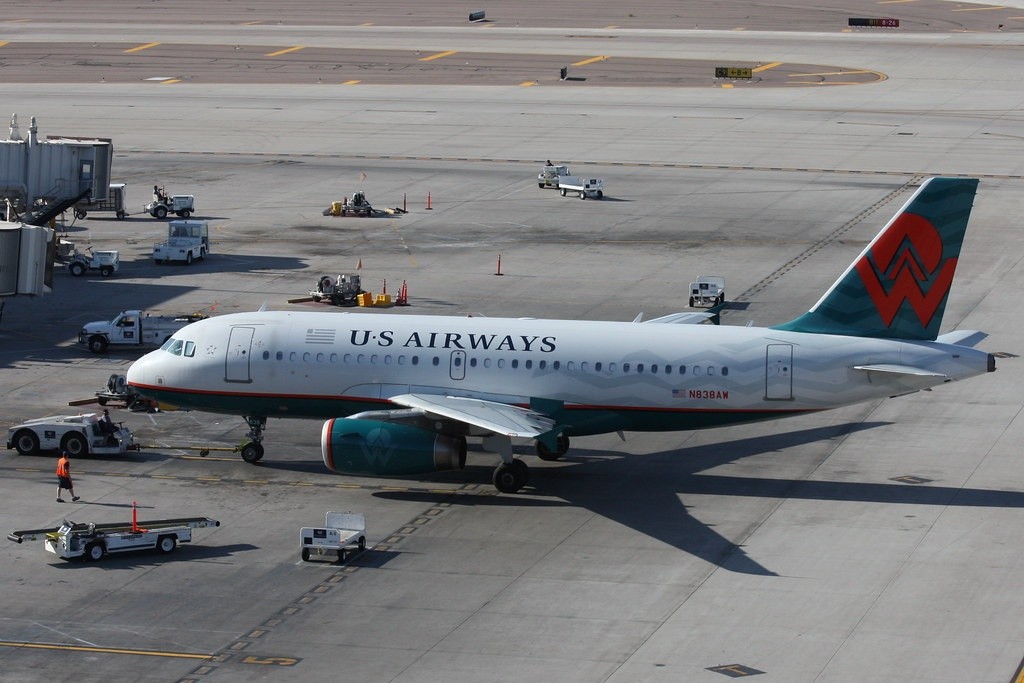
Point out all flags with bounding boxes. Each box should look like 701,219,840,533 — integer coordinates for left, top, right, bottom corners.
355,258,361,270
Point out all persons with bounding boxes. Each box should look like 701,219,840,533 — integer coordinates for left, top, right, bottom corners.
153,185,168,205
545,160,553,166
57,452,80,502
100,408,119,446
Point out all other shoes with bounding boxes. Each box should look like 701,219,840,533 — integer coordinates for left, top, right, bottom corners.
56,498,64,502
72,496,81,502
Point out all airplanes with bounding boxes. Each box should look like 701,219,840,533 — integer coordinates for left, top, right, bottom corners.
126,177,996,493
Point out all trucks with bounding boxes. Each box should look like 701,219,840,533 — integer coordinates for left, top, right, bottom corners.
78,310,214,352
44,518,192,560
153,220,209,264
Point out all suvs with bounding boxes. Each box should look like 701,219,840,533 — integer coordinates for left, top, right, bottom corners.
537,164,570,189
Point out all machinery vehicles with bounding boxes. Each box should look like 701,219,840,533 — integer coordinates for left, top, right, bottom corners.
73,183,195,222
54,236,119,276
